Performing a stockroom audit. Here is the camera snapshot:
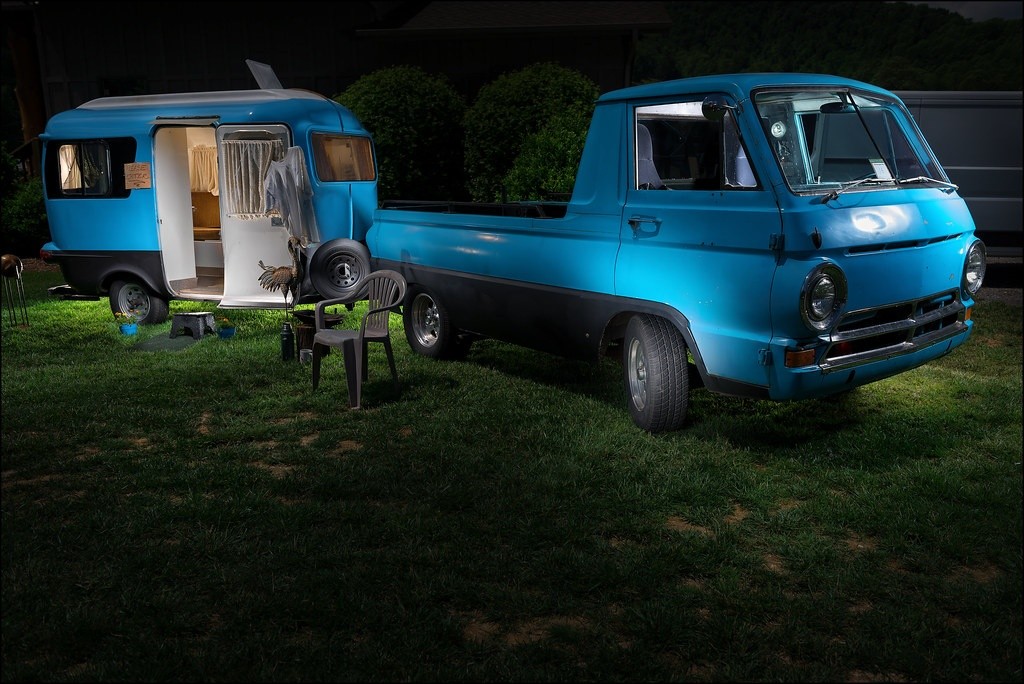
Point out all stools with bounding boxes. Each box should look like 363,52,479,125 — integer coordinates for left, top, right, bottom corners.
172,311,215,340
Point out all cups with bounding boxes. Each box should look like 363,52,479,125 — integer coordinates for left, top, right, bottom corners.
300,349,312,364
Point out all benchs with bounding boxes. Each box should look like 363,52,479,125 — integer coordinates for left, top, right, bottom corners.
191,191,221,241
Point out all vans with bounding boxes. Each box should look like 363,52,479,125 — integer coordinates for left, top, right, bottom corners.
35,58,379,327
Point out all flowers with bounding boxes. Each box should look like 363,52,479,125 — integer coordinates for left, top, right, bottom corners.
221,318,235,328
115,311,142,325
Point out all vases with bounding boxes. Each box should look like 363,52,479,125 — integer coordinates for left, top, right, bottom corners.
219,325,235,339
120,323,137,334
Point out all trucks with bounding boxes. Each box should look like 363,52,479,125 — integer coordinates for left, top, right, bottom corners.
372,75,992,434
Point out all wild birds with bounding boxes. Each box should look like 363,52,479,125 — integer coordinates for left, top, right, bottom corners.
258,236,305,300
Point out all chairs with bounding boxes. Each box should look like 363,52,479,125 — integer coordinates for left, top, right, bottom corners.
638,124,675,191
726,115,772,188
311,270,407,409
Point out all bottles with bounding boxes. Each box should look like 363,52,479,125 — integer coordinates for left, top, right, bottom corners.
280,321,294,362
